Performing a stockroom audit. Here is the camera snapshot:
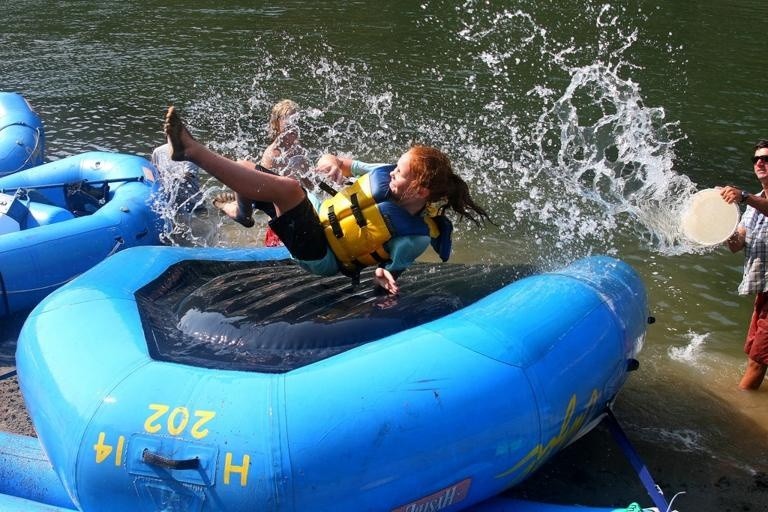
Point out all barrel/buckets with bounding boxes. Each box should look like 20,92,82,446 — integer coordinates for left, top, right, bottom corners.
682,188,742,246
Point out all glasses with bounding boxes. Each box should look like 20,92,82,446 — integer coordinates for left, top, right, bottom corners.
751,155,768,164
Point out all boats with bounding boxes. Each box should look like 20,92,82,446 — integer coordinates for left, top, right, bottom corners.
0,149,174,322
0,90,46,179
13,245,657,511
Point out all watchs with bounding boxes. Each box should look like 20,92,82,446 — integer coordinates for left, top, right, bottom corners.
740,189,749,204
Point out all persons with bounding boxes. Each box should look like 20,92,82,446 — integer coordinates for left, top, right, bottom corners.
260,98,324,248
715,138,768,392
161,106,502,298
372,294,400,310
151,141,208,213
